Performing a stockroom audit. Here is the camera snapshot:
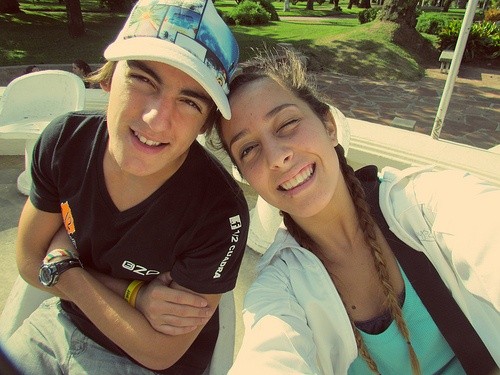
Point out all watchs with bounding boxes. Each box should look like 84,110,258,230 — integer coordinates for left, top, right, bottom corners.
34,256,81,287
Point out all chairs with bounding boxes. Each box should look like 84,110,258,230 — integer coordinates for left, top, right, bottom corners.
0,271,234,375
0,69,86,195
232,103,350,254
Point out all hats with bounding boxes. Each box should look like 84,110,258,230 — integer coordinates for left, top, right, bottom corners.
104,0,240,120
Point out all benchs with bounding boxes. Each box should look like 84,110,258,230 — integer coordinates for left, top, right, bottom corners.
438,42,469,74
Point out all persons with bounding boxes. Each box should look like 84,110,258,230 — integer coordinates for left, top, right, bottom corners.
0,0,252,374
209,48,500,375
25,57,94,90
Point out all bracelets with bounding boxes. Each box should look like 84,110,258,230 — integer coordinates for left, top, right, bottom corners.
121,279,143,308
42,249,77,261
